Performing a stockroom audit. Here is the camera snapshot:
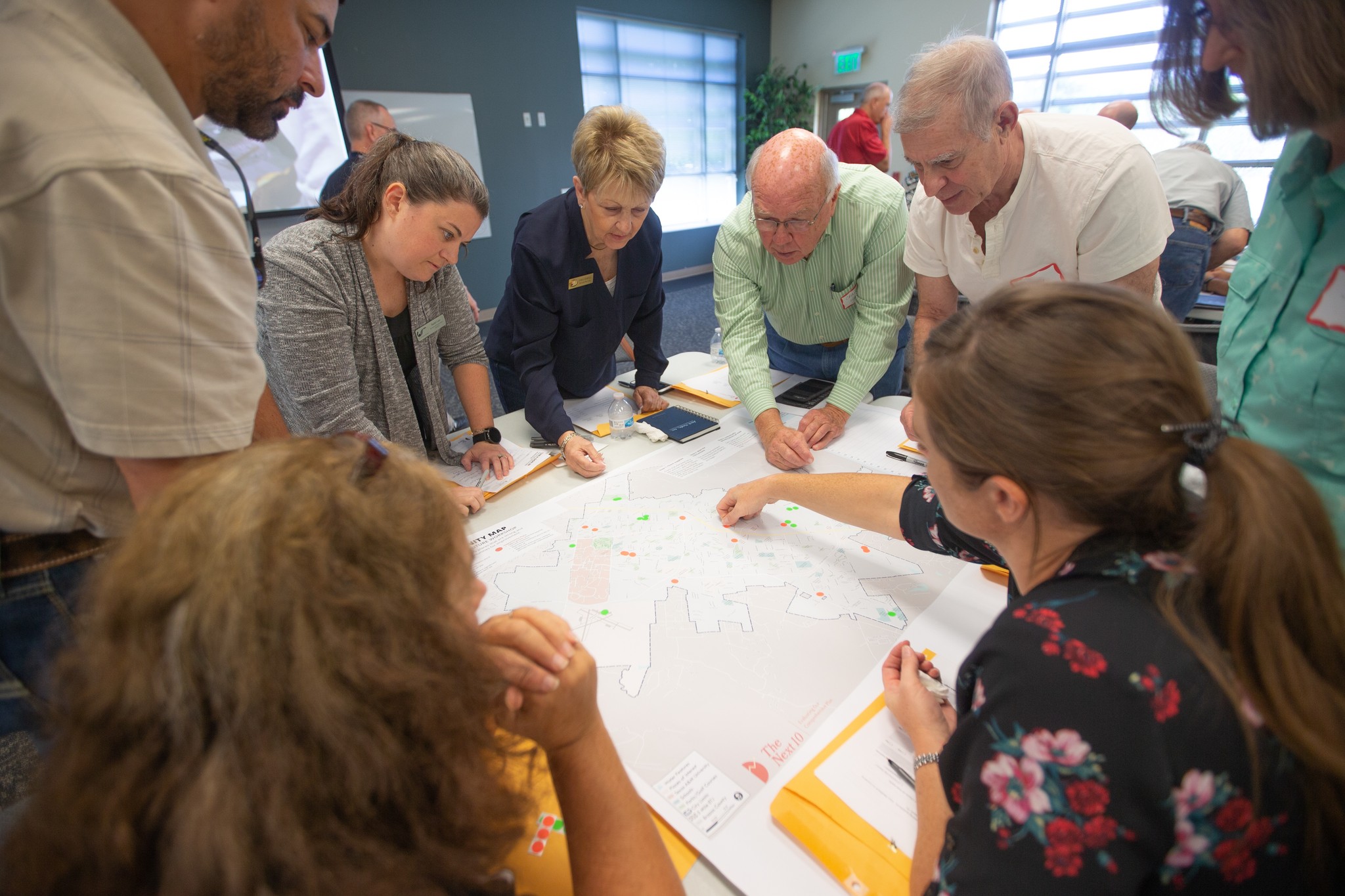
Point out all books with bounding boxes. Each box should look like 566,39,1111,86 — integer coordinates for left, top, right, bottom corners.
635,403,722,444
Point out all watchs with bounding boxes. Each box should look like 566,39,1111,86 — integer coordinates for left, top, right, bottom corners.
472,427,502,445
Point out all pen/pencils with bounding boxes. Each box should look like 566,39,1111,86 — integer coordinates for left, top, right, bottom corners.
886,756,919,787
529,434,594,447
886,450,928,468
477,469,490,488
618,380,635,388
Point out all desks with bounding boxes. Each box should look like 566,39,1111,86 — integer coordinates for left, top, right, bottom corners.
437,348,964,896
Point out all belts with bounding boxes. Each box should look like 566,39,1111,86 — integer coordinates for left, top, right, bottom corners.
1170,207,1213,231
0,529,115,576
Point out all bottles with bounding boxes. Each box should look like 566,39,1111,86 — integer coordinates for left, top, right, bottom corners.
1235,245,1248,260
608,392,635,440
710,327,727,364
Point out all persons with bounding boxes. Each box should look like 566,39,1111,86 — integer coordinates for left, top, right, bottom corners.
1097,102,1138,130
0,433,686,896
711,128,912,471
0,0,347,807
318,100,480,431
249,129,516,522
827,81,892,176
1146,140,1256,325
484,107,670,480
891,36,1177,454
719,281,1345,896
1145,0,1345,545
1200,266,1233,297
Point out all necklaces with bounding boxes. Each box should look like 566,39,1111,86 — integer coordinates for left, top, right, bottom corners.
588,242,607,250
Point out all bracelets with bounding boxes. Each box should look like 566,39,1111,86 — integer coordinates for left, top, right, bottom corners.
1202,277,1215,292
560,432,584,463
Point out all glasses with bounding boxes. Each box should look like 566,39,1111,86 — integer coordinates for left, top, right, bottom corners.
197,131,267,290
748,195,831,231
1189,9,1243,54
334,428,389,489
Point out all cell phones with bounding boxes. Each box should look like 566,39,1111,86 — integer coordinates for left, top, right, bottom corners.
630,380,674,395
782,379,834,403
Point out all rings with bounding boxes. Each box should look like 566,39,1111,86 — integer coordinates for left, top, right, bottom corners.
478,308,481,313
498,454,507,459
